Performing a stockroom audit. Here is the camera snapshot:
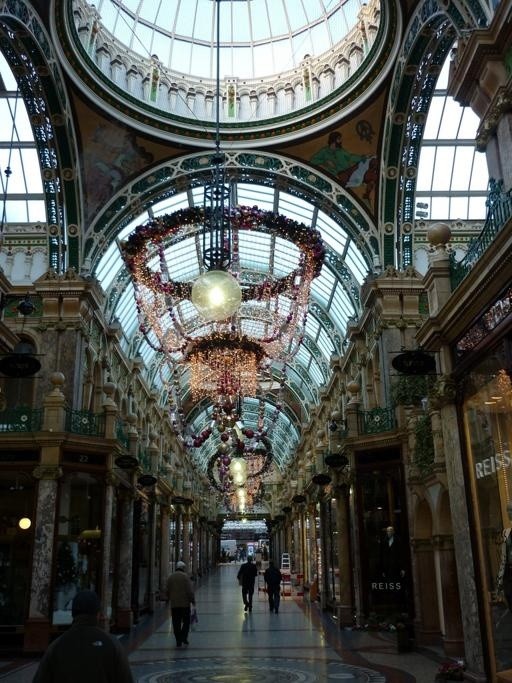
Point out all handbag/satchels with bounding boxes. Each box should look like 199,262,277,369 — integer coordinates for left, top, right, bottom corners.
191,608,198,632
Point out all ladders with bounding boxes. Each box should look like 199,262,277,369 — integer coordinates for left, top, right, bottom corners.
281,552,292,586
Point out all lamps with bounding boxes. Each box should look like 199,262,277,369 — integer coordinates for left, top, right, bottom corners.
186,1,246,324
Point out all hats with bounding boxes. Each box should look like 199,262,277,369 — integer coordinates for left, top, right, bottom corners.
177,561,185,568
72,591,99,617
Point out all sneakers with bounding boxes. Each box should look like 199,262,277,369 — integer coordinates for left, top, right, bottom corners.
244,603,252,612
270,604,278,613
176,639,182,647
181,638,189,644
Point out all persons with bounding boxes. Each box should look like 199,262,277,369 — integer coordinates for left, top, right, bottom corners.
31,590,132,682
375,525,407,615
497,504,512,610
163,561,197,649
264,561,283,614
235,557,257,612
